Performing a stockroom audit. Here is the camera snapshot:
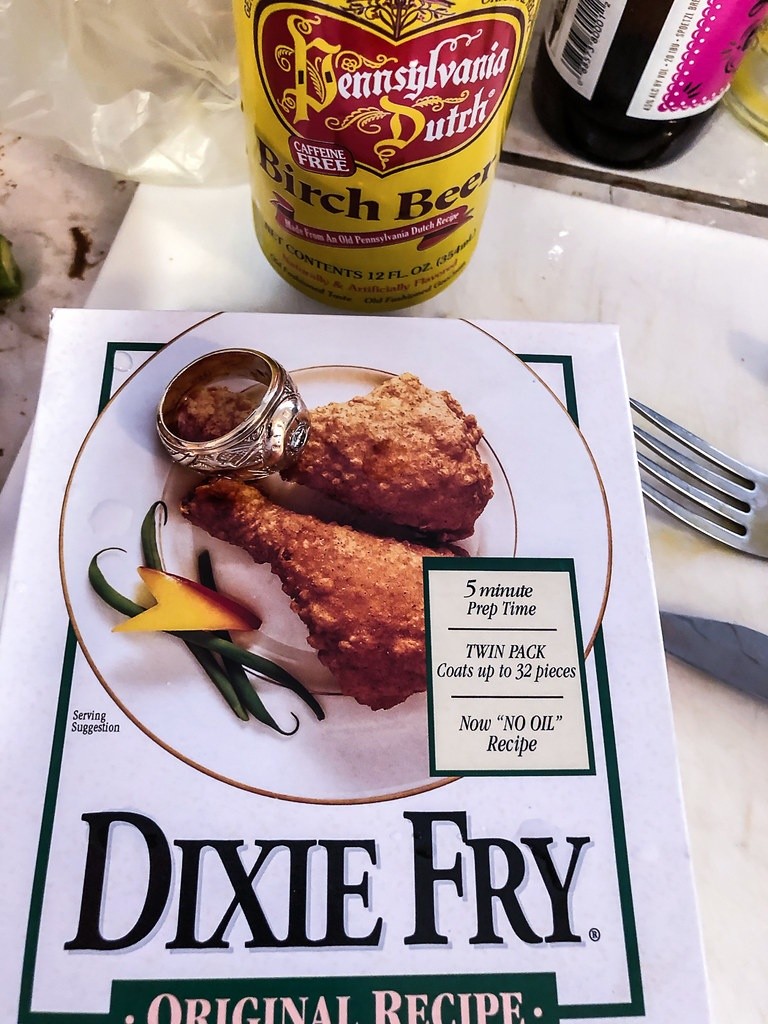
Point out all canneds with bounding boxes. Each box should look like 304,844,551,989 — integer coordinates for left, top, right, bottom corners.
233,0,541,309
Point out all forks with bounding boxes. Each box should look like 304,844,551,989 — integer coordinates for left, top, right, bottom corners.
629,397,768,560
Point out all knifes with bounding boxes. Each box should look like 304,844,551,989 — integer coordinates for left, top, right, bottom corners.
659,609,768,702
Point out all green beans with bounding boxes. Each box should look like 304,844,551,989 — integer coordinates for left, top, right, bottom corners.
88,502,325,734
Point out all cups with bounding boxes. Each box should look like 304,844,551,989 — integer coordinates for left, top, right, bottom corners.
156,347,313,488
720,16,768,145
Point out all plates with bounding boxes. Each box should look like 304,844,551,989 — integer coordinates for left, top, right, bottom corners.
57,311,614,806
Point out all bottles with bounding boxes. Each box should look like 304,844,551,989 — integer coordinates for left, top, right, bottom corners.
529,0,768,169
231,0,541,313
